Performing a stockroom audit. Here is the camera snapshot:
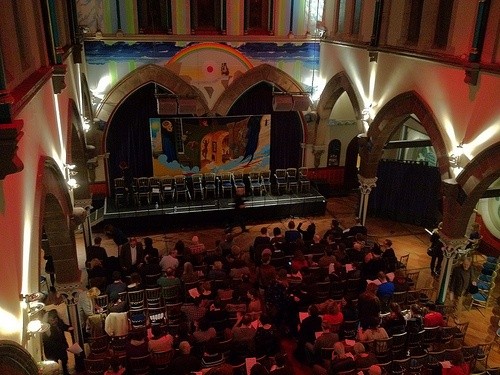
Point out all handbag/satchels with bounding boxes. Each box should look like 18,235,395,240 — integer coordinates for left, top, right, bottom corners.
427,247,433,256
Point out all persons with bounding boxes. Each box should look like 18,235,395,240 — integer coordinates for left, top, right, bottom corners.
430,222,446,277
42,309,74,375
448,257,477,325
464,222,480,250
230,188,250,233
77,219,483,375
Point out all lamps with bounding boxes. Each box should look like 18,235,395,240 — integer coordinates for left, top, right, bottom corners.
272,95,293,111
18,290,51,347
177,98,197,114
157,98,177,114
292,95,310,111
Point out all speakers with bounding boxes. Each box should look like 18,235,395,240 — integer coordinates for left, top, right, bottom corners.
291,93,310,110
272,94,293,111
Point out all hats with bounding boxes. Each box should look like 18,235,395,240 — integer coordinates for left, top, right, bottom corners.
192,236,199,242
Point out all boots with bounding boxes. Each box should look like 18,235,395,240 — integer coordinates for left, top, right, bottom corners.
431,265,441,276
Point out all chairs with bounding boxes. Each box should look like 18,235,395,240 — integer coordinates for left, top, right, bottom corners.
468,255,500,318
84,273,276,375
113,178,128,208
220,172,233,196
322,304,500,375
248,170,272,196
287,168,298,192
191,174,204,201
306,254,420,297
161,176,175,201
150,177,161,205
175,175,188,203
298,167,311,192
204,173,216,201
233,171,245,196
138,177,150,205
274,169,287,195
